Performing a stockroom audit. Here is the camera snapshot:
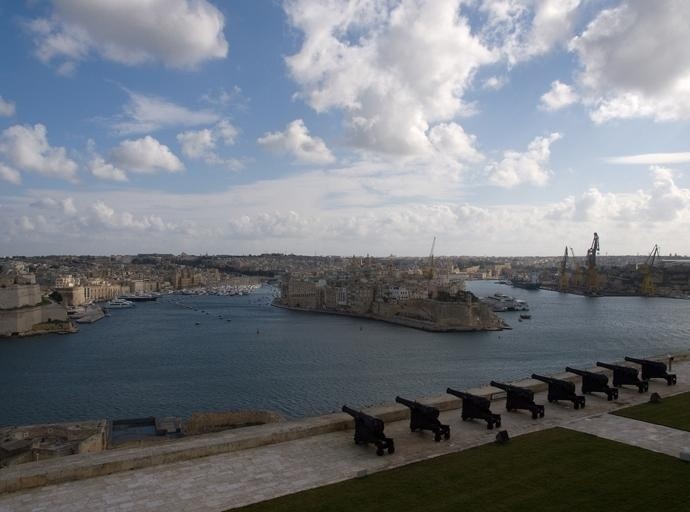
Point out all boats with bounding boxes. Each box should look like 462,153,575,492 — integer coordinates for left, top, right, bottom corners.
480,291,531,312
175,285,256,296
118,294,160,302
510,273,542,290
105,298,135,308
